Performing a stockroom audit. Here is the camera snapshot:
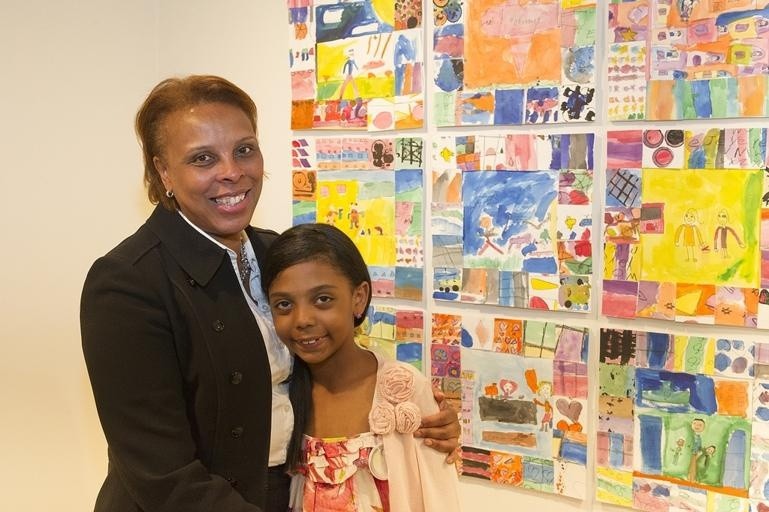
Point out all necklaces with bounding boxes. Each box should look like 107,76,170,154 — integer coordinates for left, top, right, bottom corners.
237,234,252,283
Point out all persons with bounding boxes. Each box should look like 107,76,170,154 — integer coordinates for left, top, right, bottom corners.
261,224,463,510
81,75,301,512
